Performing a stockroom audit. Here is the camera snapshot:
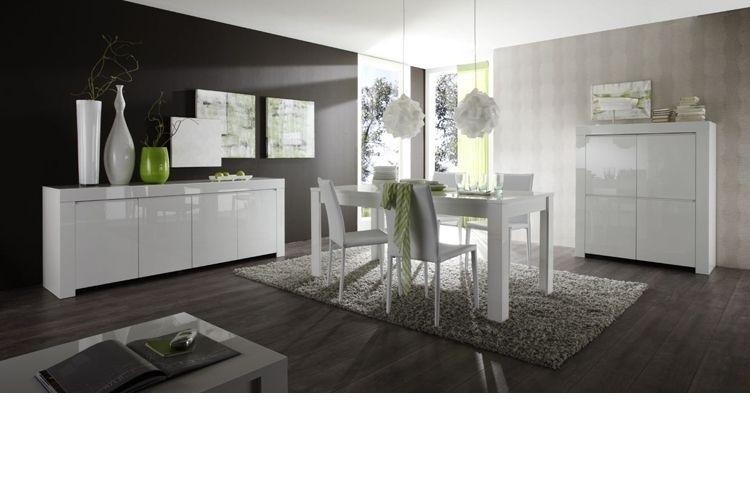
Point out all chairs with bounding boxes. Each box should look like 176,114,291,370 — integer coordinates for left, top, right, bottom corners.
380,186,481,327
373,166,399,182
317,176,387,305
463,173,534,269
432,172,463,243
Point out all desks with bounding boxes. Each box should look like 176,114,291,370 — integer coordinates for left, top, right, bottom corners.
309,184,554,322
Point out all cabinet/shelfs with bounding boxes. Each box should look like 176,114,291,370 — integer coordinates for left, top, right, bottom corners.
237,177,285,260
139,184,192,278
574,121,718,276
42,186,139,299
191,184,237,269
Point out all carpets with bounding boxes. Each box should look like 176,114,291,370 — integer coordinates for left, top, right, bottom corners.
232,240,649,371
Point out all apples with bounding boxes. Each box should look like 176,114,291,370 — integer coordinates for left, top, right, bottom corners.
237,171,245,176
216,172,229,179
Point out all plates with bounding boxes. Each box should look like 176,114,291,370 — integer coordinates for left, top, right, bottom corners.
651,95,707,122
428,180,450,193
209,175,252,182
371,163,399,185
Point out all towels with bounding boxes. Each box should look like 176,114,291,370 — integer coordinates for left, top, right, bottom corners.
380,182,414,297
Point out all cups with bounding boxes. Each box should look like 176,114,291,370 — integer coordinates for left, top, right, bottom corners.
454,170,504,196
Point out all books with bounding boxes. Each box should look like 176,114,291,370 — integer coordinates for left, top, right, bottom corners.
652,97,705,122
35,328,241,393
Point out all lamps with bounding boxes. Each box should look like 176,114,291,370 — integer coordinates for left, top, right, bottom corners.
599,98,638,124
383,0,425,139
454,0,499,139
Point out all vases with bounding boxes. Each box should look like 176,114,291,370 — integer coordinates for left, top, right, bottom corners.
140,146,169,183
76,100,102,184
103,83,136,185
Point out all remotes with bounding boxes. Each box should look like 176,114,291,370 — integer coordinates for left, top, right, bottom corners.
170,330,198,351
